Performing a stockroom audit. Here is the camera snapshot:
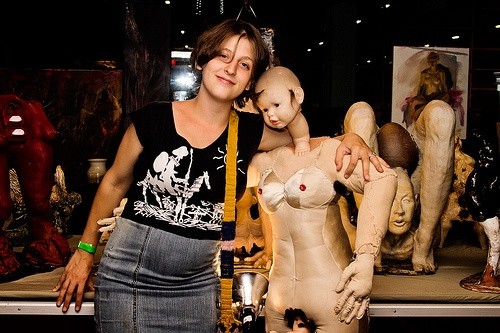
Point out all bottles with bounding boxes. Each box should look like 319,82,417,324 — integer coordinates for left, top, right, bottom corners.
87,158,106,191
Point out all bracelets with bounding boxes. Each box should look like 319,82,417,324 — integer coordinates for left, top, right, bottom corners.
77,241,97,254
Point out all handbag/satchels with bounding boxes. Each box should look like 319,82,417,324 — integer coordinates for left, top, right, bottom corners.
217,317,241,333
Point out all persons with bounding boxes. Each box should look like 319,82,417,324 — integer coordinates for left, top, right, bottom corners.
338,99,456,275
53,19,390,333
245,66,399,333
